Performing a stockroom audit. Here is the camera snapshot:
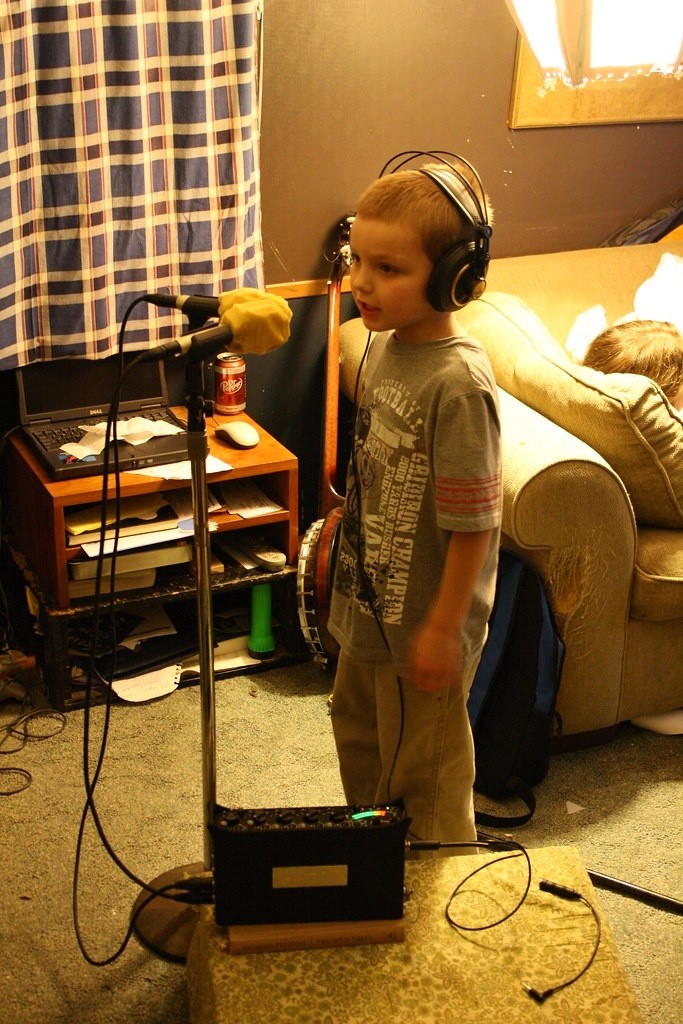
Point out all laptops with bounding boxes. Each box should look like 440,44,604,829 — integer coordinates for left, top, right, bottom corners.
17,350,212,479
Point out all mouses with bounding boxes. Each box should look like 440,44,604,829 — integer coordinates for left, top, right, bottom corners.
213,421,259,449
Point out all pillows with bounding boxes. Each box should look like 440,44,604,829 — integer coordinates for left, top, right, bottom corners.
455,289,683,528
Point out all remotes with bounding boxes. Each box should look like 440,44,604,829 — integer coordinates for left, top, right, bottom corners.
224,533,287,571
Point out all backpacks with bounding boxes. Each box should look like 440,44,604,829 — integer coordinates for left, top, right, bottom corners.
466,547,568,828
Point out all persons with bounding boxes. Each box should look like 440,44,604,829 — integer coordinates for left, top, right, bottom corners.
583,320,683,412
330,163,502,860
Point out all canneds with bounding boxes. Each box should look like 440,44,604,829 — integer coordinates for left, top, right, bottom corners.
213,352,246,414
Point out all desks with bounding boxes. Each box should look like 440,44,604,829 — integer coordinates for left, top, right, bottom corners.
184,846,642,1024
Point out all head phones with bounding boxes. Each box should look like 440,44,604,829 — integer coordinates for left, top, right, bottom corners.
378,151,494,312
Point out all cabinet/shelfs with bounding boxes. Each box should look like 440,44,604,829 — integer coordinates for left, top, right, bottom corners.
3,403,301,711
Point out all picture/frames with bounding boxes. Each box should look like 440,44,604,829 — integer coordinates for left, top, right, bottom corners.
509,1,683,134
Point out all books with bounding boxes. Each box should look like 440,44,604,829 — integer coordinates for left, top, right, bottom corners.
64,493,180,658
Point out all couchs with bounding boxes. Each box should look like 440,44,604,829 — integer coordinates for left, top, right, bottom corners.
339,224,683,752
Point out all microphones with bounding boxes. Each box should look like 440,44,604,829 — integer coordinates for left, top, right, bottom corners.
134,287,294,363
